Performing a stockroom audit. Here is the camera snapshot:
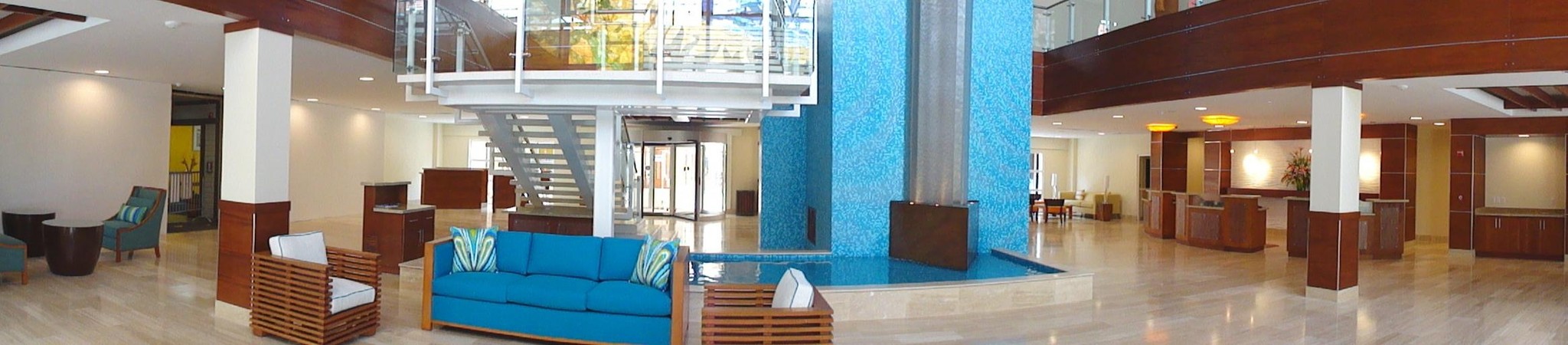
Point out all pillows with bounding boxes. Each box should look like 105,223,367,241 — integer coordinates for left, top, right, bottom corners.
268,230,329,266
771,267,815,309
115,196,156,225
1074,189,1085,200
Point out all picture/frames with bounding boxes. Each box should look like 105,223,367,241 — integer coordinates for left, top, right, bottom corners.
192,125,202,151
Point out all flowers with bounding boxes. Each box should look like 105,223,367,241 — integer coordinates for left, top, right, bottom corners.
1281,147,1312,191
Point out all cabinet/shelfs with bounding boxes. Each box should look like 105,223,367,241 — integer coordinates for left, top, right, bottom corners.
360,181,437,275
1473,207,1565,262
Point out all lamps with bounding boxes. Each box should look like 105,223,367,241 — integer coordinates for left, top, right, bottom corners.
1199,114,1241,126
1146,123,1179,132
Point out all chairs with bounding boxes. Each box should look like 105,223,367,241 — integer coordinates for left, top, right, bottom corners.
248,246,382,345
0,232,27,285
700,282,835,345
1029,194,1066,226
102,186,167,263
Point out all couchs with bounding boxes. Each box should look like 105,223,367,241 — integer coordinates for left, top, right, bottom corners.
420,230,690,345
1059,191,1122,221
